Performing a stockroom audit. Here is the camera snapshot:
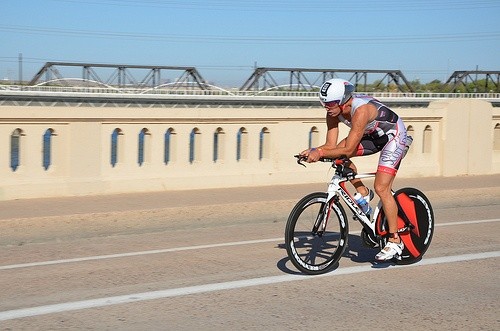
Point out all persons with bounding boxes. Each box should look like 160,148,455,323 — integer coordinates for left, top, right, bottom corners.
298,78,407,260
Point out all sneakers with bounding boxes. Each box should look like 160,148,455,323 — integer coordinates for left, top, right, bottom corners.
352,187,375,221
375,241,404,261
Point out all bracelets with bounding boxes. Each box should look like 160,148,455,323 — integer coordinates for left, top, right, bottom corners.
318,147,323,157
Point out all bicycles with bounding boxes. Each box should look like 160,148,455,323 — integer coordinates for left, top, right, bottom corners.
285,154,434,274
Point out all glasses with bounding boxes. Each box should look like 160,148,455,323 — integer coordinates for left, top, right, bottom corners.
324,102,339,107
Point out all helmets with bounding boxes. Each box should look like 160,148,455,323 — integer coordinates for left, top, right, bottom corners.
319,79,356,106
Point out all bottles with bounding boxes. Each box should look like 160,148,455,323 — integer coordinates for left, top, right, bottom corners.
354,192,372,214
